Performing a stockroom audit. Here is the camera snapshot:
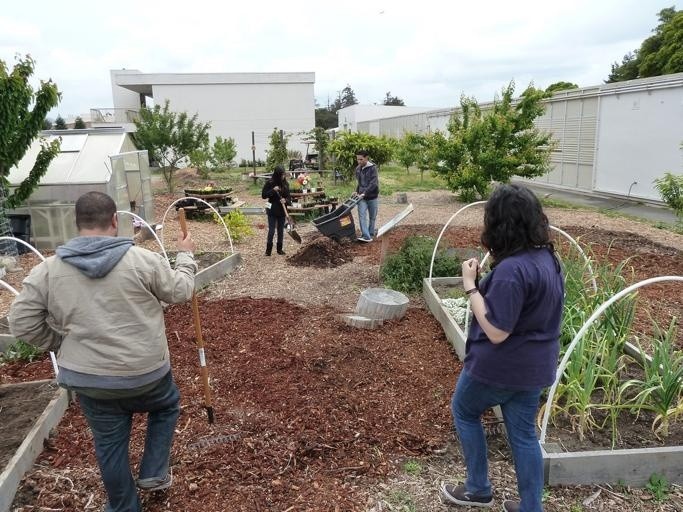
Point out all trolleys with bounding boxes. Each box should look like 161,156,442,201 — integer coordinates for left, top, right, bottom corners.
310,194,362,244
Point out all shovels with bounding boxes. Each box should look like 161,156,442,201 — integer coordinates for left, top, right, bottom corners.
274,186,301,244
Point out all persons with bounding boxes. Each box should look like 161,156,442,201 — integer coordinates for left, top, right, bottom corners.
443,185,564,512
262,166,291,256
351,150,379,242
10,191,199,512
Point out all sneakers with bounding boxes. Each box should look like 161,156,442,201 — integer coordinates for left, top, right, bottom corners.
136,474,173,492
443,483,495,507
357,237,373,242
502,499,519,512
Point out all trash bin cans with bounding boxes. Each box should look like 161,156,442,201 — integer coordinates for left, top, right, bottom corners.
241,172,249,180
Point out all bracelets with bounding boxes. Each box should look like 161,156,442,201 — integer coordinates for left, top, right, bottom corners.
466,288,478,294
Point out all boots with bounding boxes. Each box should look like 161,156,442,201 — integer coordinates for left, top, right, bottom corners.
264,242,273,256
277,242,285,255
